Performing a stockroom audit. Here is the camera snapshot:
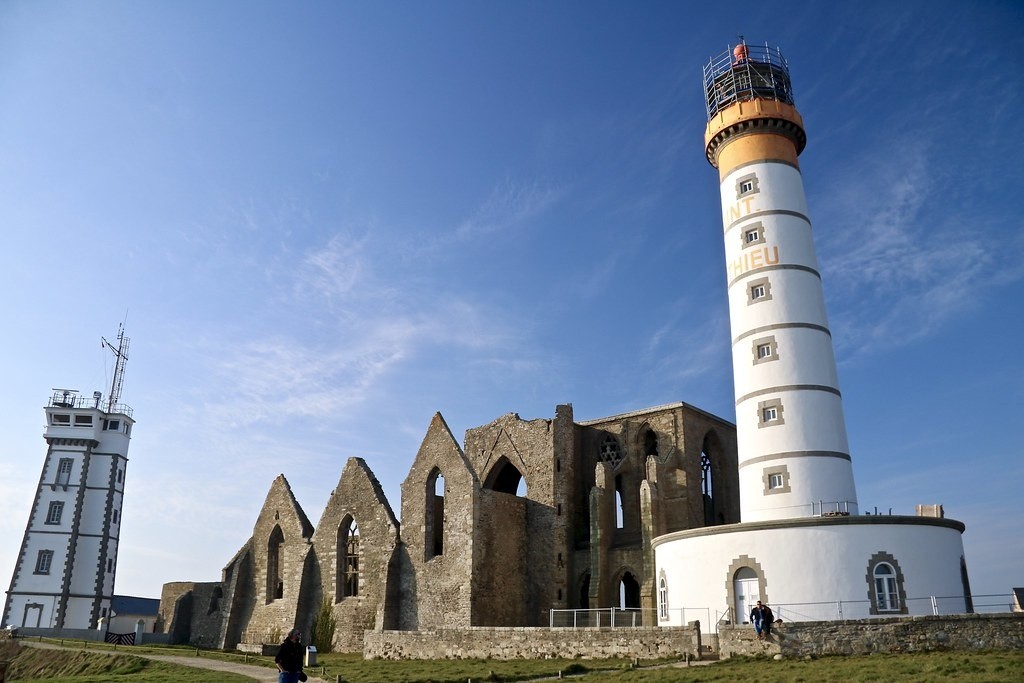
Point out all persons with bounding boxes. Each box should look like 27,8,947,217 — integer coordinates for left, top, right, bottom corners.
274,628,306,683
750,601,774,640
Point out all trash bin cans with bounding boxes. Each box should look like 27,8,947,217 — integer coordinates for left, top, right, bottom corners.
306,646,317,667
